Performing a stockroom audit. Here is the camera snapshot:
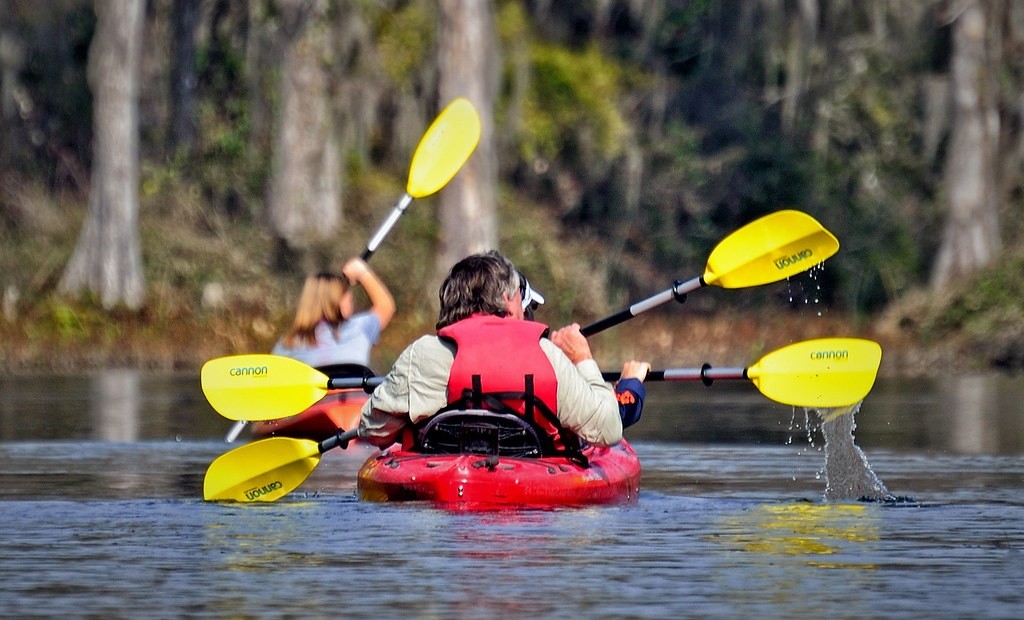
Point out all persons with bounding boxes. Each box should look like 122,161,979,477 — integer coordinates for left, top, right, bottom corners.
270,258,397,367
516,271,650,451
358,251,624,454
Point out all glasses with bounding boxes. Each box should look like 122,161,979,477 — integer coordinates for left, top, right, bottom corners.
532,301,539,310
318,271,335,280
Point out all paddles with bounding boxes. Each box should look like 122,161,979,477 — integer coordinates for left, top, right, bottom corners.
202,207,842,506
224,95,483,446
200,337,883,421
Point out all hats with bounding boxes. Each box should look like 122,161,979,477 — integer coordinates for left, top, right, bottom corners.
518,271,545,312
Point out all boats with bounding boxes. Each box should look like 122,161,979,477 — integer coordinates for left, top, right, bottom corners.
356,409,642,513
249,388,368,445
